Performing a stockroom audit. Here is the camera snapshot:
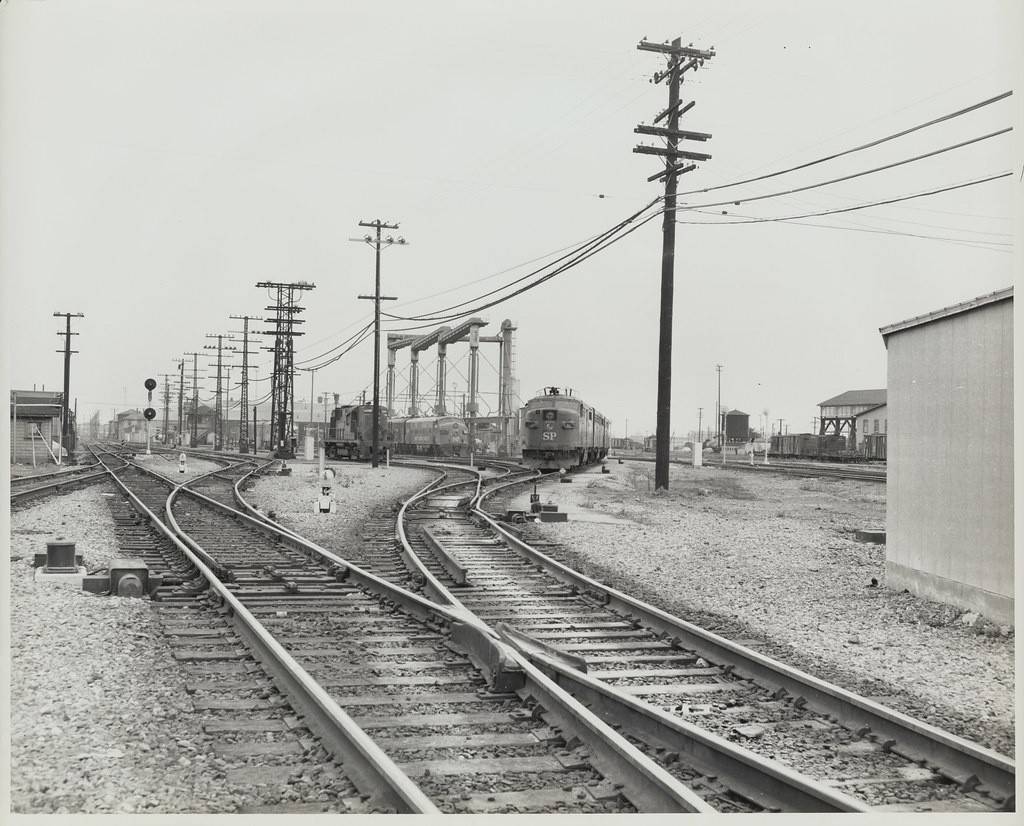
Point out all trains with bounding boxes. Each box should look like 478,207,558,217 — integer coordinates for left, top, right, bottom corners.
521,395,610,473
324,404,468,462
